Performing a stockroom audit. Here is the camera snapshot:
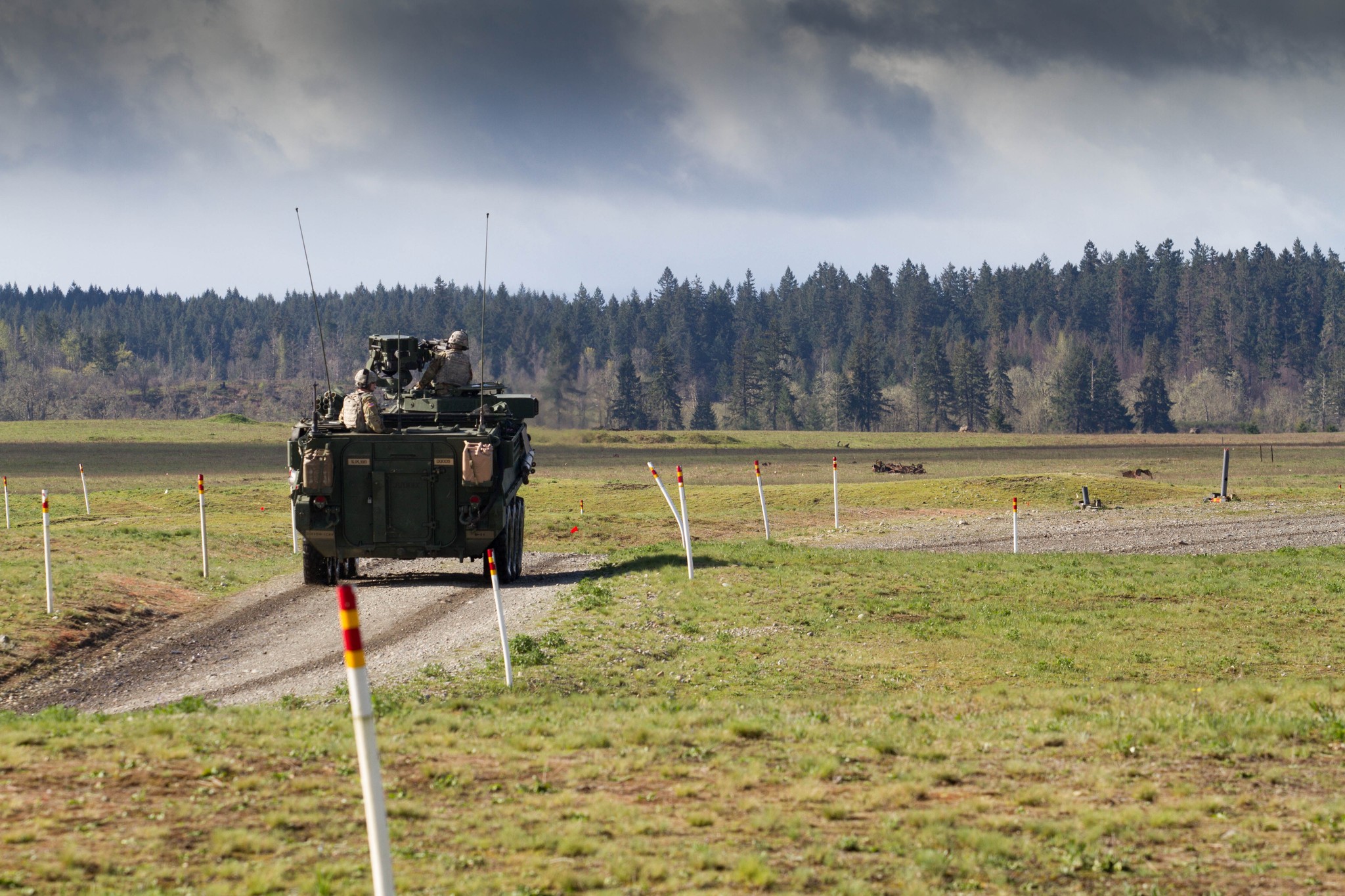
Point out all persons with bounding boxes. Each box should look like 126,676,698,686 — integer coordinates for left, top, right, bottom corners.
413,330,473,397
338,368,395,434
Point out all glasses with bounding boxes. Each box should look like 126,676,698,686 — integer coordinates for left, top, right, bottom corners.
371,382,376,384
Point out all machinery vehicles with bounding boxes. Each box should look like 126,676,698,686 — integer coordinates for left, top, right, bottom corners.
288,332,540,585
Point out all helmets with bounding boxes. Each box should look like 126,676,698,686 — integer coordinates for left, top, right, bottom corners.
448,330,468,347
354,369,376,384
